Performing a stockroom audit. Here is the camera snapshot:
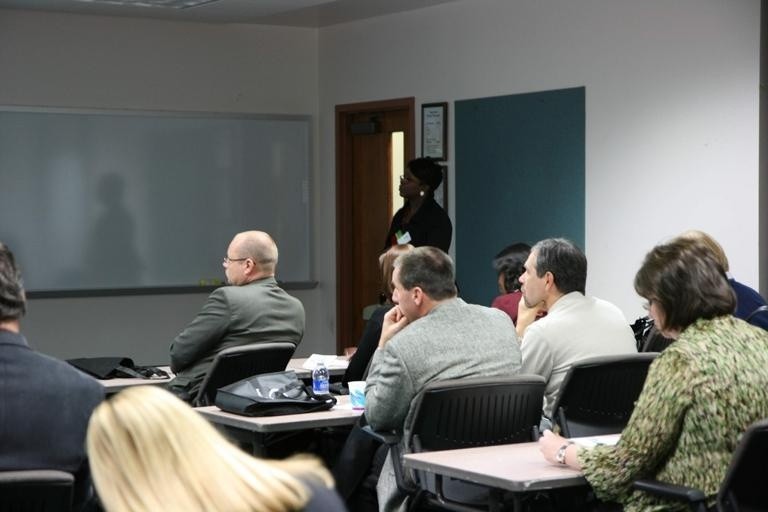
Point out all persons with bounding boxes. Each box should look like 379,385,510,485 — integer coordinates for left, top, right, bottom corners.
322,247,524,512
86,381,352,511
0,247,108,511
379,157,454,256
167,229,308,410
340,243,413,393
679,228,768,324
533,237,767,510
489,241,532,321
518,234,642,439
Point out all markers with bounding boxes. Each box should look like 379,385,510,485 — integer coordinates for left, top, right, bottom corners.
199,279,220,285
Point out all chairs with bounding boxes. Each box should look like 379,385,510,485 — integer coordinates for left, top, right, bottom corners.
1,471,74,510
192,343,296,405
362,352,768,511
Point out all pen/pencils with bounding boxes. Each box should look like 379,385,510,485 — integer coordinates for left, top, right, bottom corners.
351,407,365,411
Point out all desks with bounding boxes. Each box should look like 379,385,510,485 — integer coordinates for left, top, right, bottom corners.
97,355,362,457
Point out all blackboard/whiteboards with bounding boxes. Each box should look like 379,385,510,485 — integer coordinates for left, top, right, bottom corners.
0,106,318,300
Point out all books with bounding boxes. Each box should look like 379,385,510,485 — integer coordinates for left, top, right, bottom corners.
301,351,350,371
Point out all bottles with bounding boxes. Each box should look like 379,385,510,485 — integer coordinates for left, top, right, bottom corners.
312,362,330,396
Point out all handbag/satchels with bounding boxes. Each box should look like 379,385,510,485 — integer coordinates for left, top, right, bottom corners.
213,368,338,418
61,355,173,383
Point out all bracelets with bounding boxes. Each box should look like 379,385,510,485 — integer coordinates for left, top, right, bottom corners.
553,440,576,465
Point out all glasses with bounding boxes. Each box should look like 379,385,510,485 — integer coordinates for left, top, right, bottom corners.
223,255,248,265
641,300,656,312
399,174,421,185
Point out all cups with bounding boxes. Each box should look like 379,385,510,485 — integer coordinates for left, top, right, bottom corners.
348,381,366,410
